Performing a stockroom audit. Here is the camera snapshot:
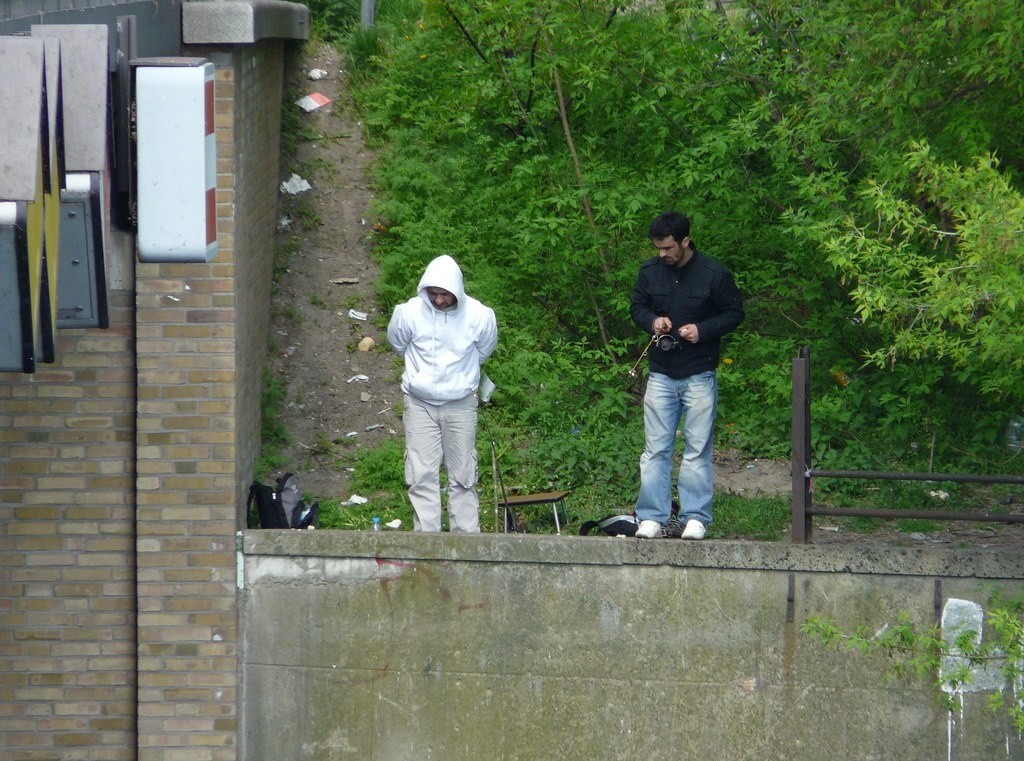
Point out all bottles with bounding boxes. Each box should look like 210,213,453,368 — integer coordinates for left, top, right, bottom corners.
370,517,383,531
297,504,312,525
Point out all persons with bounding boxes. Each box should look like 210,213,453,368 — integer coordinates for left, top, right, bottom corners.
387,254,498,533
628,211,746,540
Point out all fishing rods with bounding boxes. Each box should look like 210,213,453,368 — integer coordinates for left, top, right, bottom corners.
629,324,665,378
477,401,514,533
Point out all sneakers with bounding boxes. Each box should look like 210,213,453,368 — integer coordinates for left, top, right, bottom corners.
680,519,706,540
635,520,664,539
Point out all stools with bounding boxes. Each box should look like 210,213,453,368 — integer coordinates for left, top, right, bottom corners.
498,491,569,535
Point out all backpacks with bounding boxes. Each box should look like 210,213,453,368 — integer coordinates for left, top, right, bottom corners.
578,513,640,536
245,472,322,532
